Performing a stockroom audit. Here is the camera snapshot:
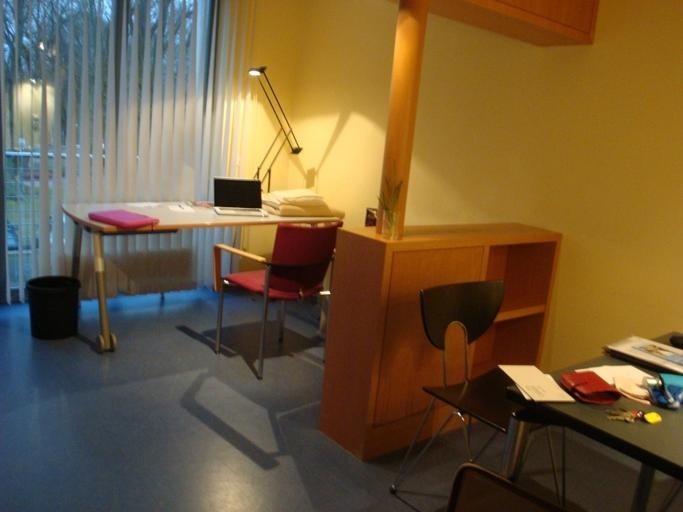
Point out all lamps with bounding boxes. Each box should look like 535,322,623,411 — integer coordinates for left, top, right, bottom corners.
248,65,304,194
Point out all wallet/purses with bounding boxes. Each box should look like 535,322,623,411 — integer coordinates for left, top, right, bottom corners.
559,371,622,405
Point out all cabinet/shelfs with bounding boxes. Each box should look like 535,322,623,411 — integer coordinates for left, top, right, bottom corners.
319,221,565,462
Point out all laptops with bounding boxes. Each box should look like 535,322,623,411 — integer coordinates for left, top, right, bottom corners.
211,177,269,218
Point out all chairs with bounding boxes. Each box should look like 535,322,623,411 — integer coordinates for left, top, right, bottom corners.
388,279,563,494
444,462,564,511
211,221,344,382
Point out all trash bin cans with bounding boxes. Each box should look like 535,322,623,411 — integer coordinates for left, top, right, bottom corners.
26,274,81,341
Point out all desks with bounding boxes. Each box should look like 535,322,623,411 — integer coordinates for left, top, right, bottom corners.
504,331,682,511
60,200,341,353
